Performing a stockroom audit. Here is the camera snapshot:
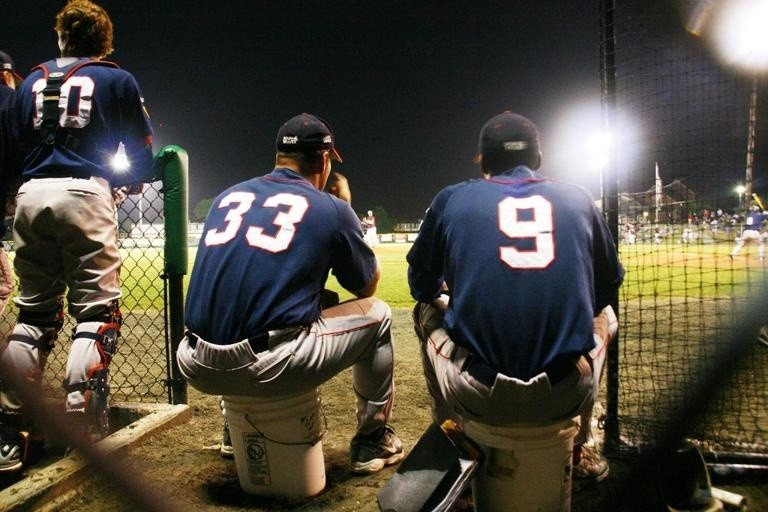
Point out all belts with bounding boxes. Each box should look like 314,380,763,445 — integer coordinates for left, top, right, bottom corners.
186,332,305,360
466,362,589,393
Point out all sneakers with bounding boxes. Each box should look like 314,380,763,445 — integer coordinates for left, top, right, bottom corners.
220,425,234,456
349,429,404,473
574,456,610,486
0,434,24,471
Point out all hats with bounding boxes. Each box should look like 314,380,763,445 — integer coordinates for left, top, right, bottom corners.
0,52,24,83
277,112,342,163
473,111,539,160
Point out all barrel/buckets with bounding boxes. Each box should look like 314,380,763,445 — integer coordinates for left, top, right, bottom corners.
220,392,328,499
460,418,581,512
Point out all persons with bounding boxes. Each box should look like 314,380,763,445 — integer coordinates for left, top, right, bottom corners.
176,112,404,473
361,210,376,249
406,110,625,488
0,0,153,472
617,205,768,262
